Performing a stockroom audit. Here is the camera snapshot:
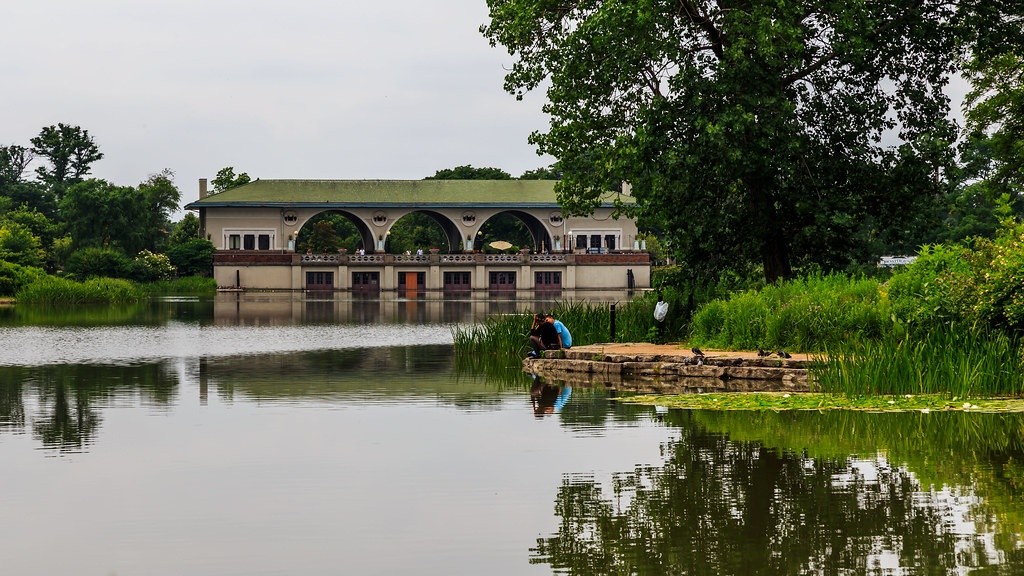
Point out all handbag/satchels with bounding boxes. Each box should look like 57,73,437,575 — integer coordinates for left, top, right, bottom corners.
654,301,668,323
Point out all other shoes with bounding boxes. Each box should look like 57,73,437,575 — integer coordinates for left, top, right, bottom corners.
529,355,541,359
526,351,536,356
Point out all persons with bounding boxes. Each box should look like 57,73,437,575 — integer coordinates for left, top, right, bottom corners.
527,313,572,359
403,247,423,256
531,375,572,420
355,247,365,256
